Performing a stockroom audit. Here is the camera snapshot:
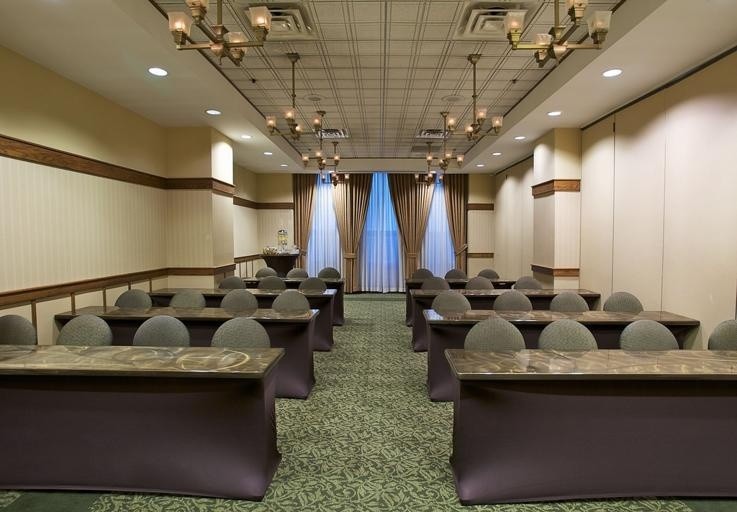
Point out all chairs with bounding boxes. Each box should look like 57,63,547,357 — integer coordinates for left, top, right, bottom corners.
0,266,347,503
402,267,735,508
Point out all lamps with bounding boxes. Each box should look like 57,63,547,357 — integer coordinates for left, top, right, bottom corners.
166,0,351,189
412,1,615,189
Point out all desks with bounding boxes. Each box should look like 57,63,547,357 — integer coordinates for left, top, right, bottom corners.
258,253,300,278
